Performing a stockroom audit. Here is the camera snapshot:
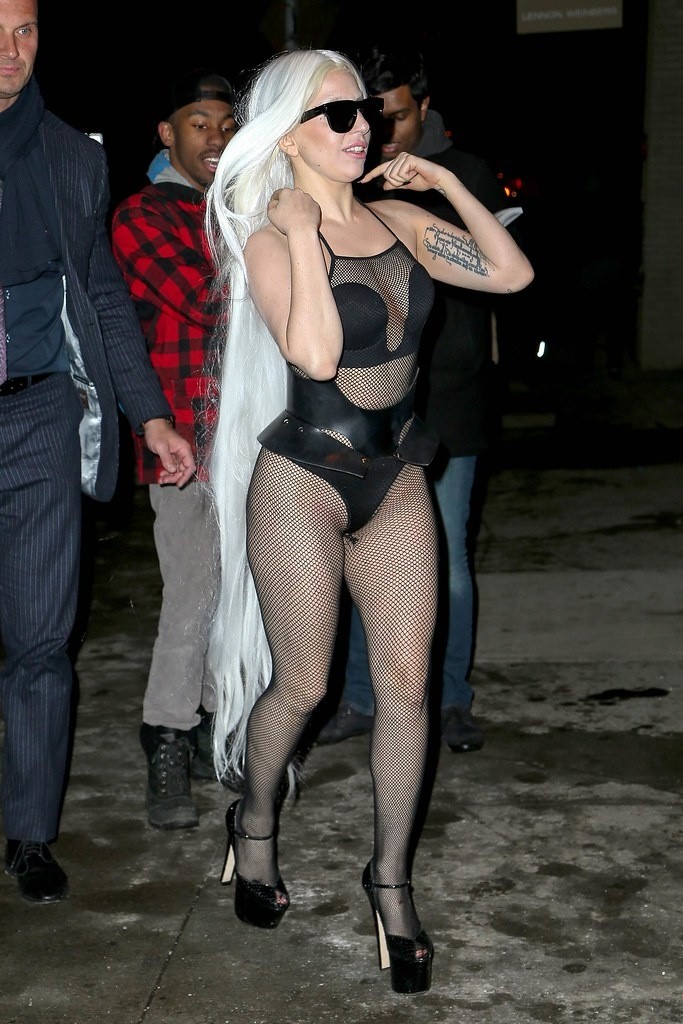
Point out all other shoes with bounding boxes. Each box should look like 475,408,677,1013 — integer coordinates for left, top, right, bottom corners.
438,706,485,753
312,701,375,745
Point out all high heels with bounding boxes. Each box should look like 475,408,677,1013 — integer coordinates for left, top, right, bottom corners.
220,799,290,930
362,857,433,994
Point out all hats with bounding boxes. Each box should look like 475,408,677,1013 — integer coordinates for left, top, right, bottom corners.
152,68,236,158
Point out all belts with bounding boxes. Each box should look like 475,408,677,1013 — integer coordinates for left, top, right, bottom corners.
0,372,54,397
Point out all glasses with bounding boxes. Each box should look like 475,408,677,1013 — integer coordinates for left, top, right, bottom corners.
300,97,384,133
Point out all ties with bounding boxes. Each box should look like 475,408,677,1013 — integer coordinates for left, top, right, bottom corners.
0,179,6,386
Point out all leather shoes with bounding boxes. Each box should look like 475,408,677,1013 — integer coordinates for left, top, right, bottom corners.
4,838,67,904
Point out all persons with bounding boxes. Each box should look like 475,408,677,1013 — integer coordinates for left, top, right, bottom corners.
312,50,510,753
202,48,534,991
1,0,198,904
109,67,271,832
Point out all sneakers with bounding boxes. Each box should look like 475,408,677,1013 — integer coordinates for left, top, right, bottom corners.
192,740,243,793
145,733,199,830
